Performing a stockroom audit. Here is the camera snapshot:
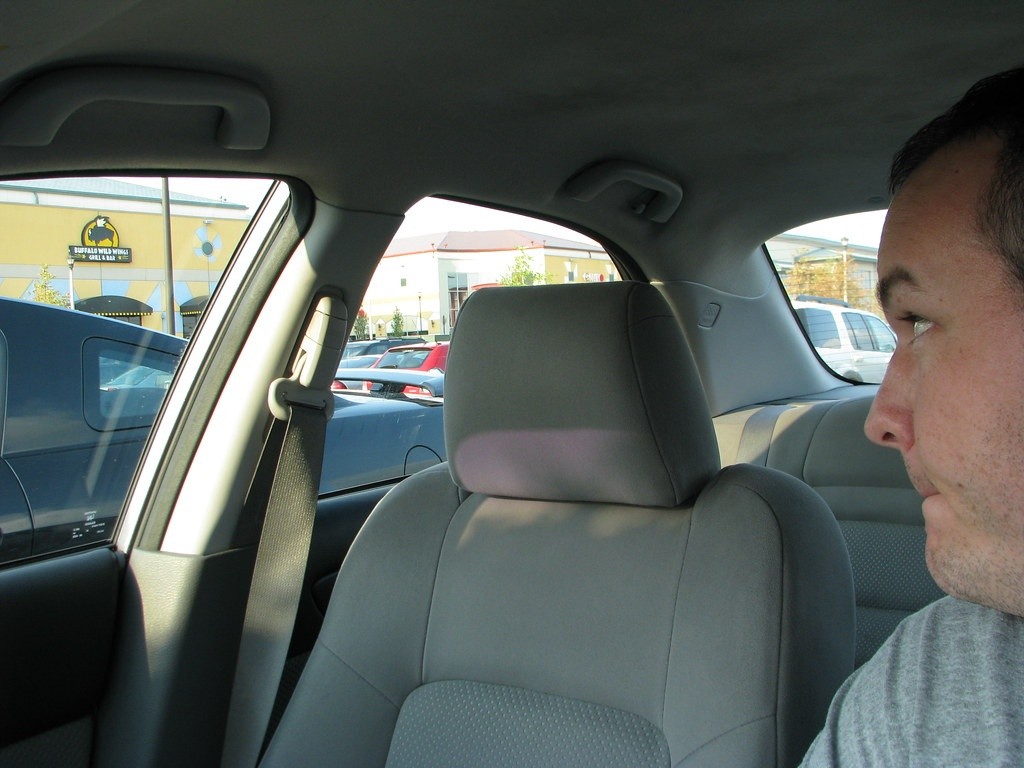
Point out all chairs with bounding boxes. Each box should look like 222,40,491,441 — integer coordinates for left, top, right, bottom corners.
710,400,954,672
262,280,858,768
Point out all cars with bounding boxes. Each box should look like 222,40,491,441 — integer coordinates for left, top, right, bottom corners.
0,296,446,572
99,335,451,399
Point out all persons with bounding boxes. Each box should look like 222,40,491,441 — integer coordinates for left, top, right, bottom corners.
795,63,1024,766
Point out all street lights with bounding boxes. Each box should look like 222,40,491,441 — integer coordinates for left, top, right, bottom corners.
448,271,461,312
840,236,852,302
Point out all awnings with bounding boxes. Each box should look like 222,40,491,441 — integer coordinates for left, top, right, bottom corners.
179,295,209,315
75,295,153,315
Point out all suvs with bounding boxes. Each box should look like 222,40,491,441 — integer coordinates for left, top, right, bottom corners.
787,293,899,385
